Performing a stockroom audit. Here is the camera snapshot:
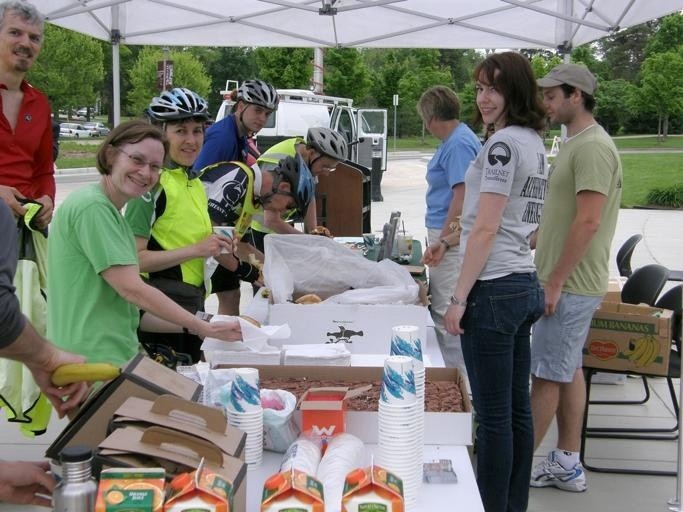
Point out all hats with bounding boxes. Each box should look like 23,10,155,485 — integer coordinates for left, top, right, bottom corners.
534,60,600,97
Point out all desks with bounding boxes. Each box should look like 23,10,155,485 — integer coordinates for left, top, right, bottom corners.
52,231,484,512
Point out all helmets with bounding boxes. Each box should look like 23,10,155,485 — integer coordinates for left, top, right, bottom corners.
275,150,318,212
306,126,349,162
236,78,278,111
146,86,211,124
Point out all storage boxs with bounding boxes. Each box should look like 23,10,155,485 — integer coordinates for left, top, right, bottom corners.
581,300,673,376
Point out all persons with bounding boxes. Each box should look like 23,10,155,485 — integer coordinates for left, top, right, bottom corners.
528,66,624,494
249,127,349,298
416,86,483,400
46,122,244,393
442,53,545,512
122,87,244,371
1,198,93,508
197,153,315,283
193,77,279,317
0,1,55,238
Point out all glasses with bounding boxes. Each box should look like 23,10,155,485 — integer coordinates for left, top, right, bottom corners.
116,145,166,175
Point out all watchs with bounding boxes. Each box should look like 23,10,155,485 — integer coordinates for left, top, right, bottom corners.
450,296,467,306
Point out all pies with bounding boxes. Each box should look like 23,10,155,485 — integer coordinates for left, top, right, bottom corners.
237,315,261,327
292,294,321,304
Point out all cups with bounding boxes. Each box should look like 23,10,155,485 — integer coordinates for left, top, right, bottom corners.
226,325,429,511
212,226,236,255
362,230,422,264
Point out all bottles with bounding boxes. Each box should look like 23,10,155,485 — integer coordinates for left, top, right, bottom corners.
50,446,100,511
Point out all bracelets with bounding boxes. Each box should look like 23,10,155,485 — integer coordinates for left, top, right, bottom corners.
183,327,188,335
440,238,450,250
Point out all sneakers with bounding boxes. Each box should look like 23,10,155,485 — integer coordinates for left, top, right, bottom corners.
530,451,589,495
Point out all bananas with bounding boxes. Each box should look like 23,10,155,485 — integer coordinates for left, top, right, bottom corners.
51,363,122,386
623,334,661,368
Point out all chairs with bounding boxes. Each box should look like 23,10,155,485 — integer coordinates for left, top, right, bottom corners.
583,264,680,433
616,232,643,277
579,284,683,475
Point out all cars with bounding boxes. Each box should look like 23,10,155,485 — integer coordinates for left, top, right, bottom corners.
77,106,95,118
58,122,110,139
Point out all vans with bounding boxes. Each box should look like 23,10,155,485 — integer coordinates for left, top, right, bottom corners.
214,87,388,203
